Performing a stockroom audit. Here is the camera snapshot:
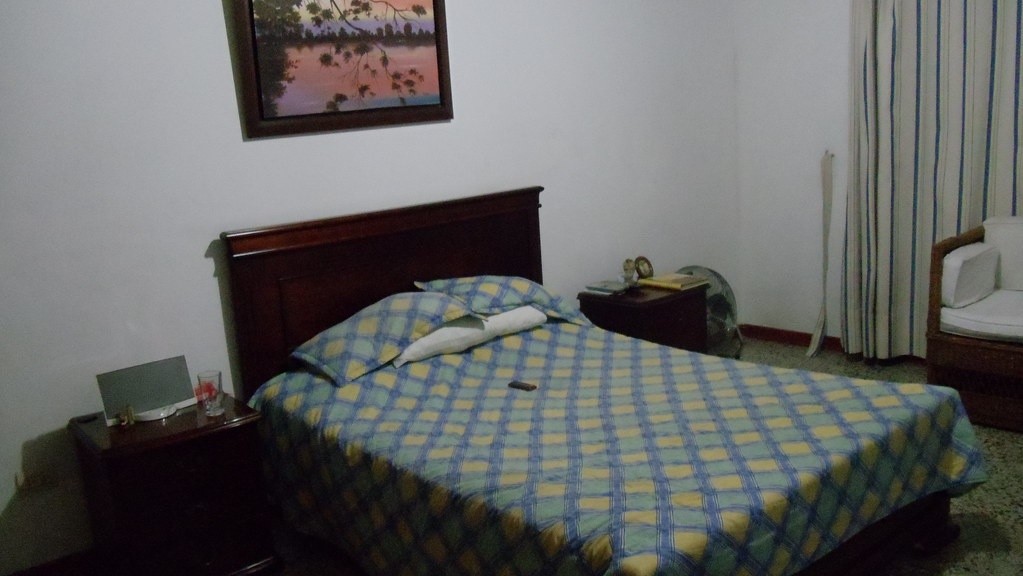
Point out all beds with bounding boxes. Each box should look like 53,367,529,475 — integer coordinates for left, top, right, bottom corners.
221,186,989,575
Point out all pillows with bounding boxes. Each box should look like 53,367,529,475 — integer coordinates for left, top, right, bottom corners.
392,304,548,369
413,274,595,329
287,291,489,387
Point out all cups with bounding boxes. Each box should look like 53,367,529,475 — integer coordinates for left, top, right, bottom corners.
197,370,224,417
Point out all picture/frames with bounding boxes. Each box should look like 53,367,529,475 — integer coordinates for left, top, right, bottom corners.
221,0,454,143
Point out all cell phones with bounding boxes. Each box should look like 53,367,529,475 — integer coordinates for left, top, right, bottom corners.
509,380,536,391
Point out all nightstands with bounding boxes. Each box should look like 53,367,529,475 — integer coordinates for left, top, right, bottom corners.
576,284,713,354
66,388,284,576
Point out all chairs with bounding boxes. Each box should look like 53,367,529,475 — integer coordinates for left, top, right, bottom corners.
924,215,1023,435
